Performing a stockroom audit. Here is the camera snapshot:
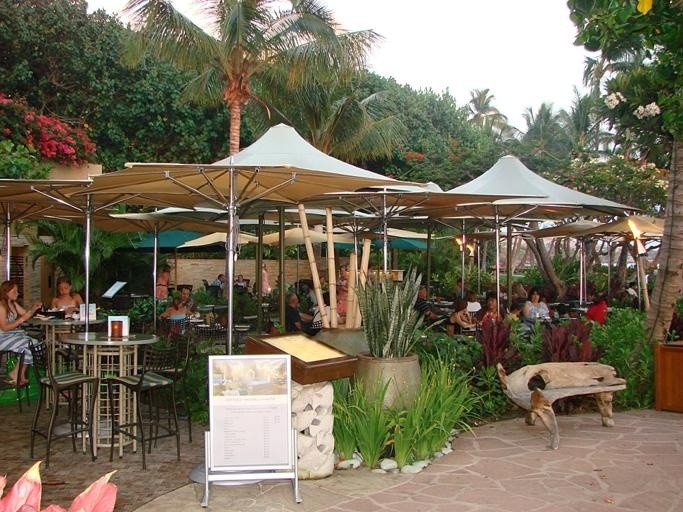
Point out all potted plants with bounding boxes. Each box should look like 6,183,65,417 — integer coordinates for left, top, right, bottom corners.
354,278,447,410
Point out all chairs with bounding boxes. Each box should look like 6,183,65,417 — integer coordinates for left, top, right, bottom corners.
1,278,297,471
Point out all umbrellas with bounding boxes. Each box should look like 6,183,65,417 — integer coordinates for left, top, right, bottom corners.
0,177,198,455
0,200,68,281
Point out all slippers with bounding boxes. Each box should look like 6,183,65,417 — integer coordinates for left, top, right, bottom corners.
4,379,29,386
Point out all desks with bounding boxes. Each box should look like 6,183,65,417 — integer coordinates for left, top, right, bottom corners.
426,296,625,336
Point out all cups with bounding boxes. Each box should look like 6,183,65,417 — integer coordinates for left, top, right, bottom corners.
110,320,122,338
